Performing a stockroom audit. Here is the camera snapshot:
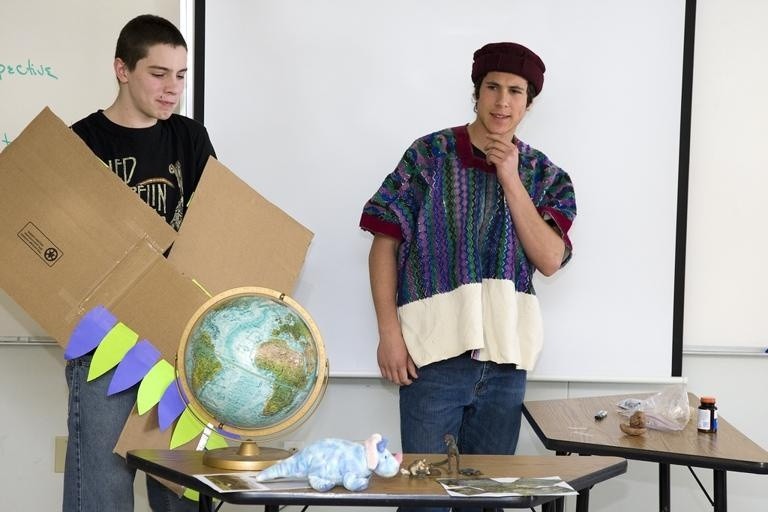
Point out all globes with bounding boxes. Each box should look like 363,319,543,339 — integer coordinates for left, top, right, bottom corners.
174,286,328,470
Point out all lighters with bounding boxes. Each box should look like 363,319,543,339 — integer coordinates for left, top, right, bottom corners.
595,410,607,420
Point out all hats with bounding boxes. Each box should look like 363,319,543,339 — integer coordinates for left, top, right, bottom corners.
472,42,545,97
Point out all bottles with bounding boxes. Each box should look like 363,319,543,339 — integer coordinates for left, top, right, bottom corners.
697,397,718,433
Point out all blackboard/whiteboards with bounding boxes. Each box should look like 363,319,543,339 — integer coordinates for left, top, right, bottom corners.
0,0,768,356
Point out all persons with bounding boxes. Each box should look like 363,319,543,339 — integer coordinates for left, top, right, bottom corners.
360,42,576,511
61,15,217,512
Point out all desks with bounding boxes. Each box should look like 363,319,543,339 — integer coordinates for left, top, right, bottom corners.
123,446,630,512
517,388,768,512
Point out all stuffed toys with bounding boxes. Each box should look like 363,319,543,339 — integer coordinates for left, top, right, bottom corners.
255,434,403,493
